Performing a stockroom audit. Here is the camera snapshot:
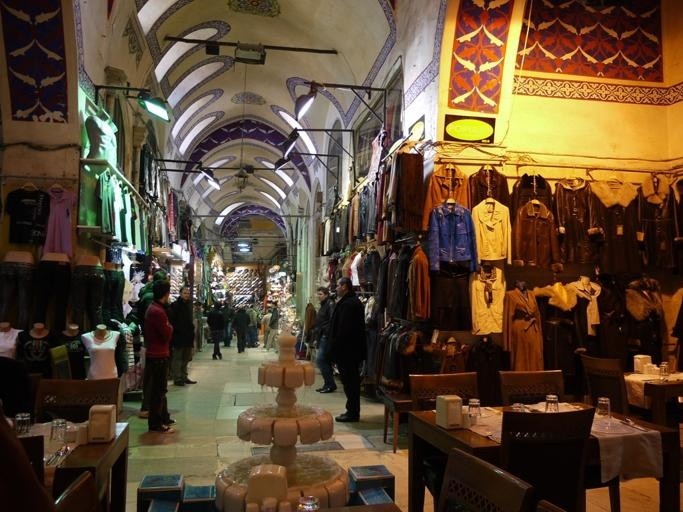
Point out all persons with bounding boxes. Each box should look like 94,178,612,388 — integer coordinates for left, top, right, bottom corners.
166,287,197,386
144,280,178,433
139,272,172,418
315,287,337,393
85,109,119,169
504,274,665,395
325,277,365,422
193,301,278,359
0,250,135,379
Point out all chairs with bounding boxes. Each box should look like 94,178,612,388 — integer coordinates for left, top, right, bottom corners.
1,415,101,512
409,372,481,511
380,357,469,452
536,499,567,512
501,406,596,512
28,374,121,510
437,447,534,511
578,352,630,416
497,370,566,408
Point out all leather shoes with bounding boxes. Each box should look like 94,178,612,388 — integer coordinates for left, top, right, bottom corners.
335,413,360,422
316,386,337,393
174,379,197,385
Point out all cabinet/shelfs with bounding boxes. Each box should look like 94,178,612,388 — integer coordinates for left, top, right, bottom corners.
79,157,150,259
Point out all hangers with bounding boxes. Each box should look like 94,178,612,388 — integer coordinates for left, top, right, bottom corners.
440,159,663,186
442,191,544,213
481,261,499,274
102,165,149,213
274,152,341,202
16,182,68,194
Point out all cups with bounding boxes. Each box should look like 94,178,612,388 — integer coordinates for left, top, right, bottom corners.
15,413,31,436
50,419,68,444
512,403,525,412
545,395,559,413
597,397,611,416
659,362,670,376
468,398,481,417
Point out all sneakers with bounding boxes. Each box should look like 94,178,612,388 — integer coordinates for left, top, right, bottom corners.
148,419,175,433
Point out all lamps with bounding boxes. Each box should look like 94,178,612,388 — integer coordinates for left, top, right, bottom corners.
165,169,221,190
294,80,388,132
282,128,357,185
399,142,421,155
94,85,172,125
